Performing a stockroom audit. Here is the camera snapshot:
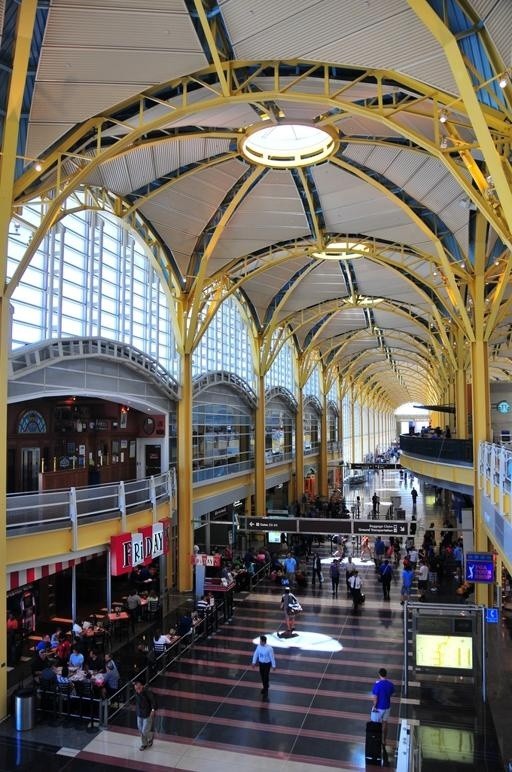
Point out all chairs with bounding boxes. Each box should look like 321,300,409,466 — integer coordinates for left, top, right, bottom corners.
33,601,208,719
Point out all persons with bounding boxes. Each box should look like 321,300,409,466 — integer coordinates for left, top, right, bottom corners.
372,493,378,513
362,517,463,605
282,552,363,609
134,681,156,750
214,547,270,584
35,620,121,697
127,588,158,611
411,488,418,503
153,593,214,653
281,587,301,632
251,635,276,700
374,424,451,481
309,497,324,518
370,668,397,747
332,534,349,558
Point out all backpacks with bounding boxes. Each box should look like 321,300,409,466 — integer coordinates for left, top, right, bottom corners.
332,566,339,579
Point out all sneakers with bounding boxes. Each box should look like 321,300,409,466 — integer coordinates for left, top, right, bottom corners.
139,735,154,751
261,688,268,696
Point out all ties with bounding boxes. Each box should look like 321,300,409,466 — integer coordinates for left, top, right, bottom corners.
354,578,356,588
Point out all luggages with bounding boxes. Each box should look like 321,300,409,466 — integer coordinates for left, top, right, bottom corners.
365,711,383,759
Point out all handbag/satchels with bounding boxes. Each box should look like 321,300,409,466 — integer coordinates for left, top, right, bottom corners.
377,574,383,581
360,593,365,603
287,601,303,615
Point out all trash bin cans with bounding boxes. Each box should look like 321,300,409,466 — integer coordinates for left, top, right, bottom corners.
13,689,36,732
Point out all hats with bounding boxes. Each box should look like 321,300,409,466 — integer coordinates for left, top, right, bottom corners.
403,559,409,565
